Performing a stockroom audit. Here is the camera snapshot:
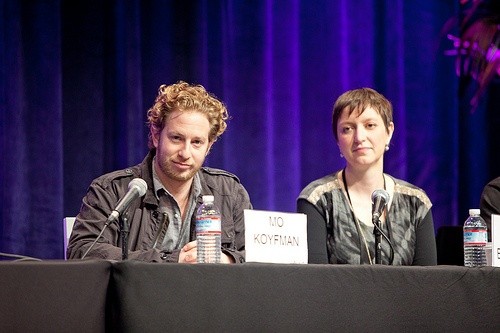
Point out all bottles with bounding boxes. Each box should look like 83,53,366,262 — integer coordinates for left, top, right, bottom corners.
195,195,222,263
463,209,487,267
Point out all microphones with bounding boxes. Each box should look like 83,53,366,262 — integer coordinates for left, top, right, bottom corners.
371,189,390,223
105,178,148,226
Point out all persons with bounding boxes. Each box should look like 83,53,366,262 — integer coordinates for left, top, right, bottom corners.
66,81,253,264
296,87,437,267
